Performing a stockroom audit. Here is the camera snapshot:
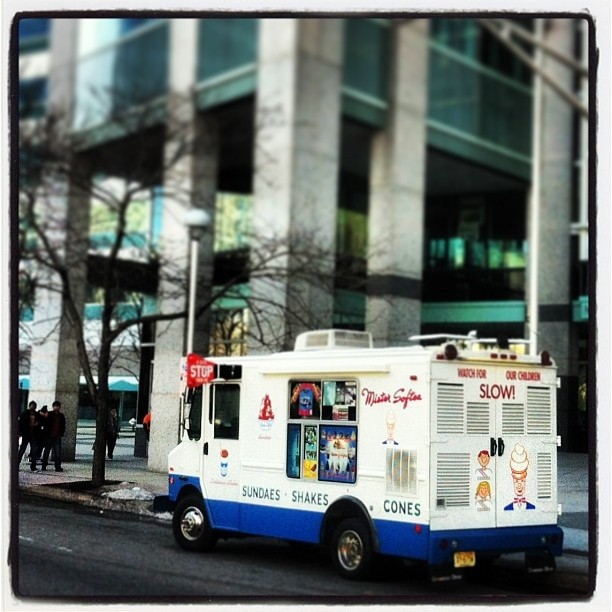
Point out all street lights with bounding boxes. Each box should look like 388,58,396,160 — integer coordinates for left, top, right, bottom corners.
184,208,210,355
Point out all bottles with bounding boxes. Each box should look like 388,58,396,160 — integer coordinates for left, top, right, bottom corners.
221,449,228,478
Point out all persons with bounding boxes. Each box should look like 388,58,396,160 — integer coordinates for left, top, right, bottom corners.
106,408,121,459
41,401,65,472
18,401,40,472
28,406,47,462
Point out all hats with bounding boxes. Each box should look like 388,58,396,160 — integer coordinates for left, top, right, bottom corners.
41,405,47,413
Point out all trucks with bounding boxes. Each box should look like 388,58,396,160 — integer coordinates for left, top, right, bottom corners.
167,328,563,583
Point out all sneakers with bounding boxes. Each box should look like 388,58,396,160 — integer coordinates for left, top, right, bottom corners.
31,467,39,473
55,467,63,472
